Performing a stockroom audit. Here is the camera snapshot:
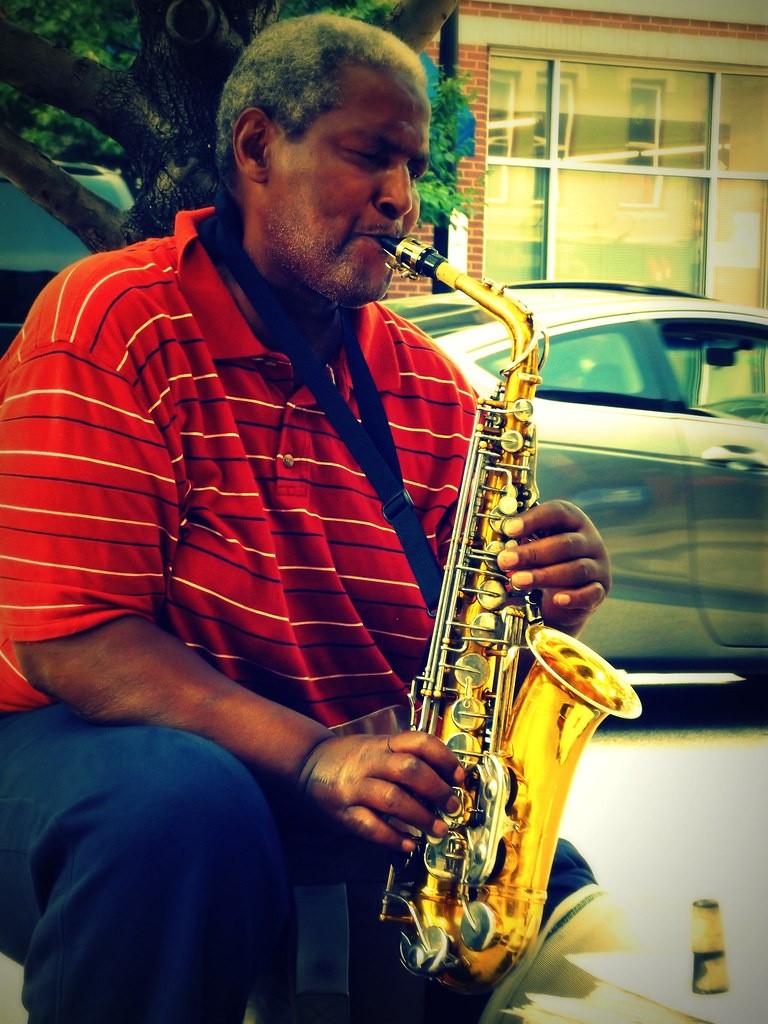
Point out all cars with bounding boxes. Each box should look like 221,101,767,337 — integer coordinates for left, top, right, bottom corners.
378,281,768,680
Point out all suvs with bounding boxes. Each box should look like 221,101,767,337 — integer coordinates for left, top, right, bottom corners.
0,160,136,357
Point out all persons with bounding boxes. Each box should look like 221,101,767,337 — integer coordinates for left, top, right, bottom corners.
1,14,609,1024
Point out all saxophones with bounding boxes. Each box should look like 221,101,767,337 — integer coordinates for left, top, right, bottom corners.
375,232,642,997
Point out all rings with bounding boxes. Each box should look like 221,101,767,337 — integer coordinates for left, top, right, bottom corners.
387,735,393,754
